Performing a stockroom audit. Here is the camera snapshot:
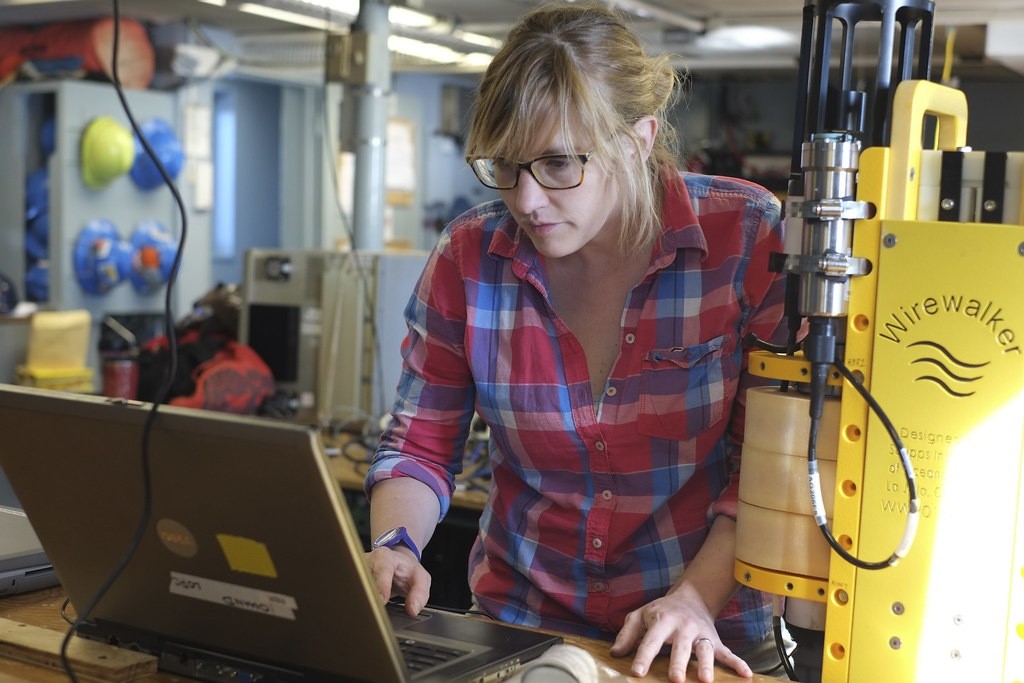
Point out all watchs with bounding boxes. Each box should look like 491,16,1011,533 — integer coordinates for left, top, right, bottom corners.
371,525,423,563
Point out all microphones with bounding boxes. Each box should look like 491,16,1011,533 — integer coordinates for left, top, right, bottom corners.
800,130,861,419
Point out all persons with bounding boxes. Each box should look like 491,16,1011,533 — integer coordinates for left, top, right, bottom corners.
688,145,745,178
358,6,796,683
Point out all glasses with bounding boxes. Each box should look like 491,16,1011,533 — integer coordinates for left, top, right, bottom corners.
465,122,627,190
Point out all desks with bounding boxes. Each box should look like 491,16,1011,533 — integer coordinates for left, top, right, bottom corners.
272,399,491,534
0,577,798,683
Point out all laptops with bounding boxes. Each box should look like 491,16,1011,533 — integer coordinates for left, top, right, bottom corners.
0,382,564,683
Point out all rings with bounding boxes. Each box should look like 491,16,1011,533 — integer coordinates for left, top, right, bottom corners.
694,637,713,646
